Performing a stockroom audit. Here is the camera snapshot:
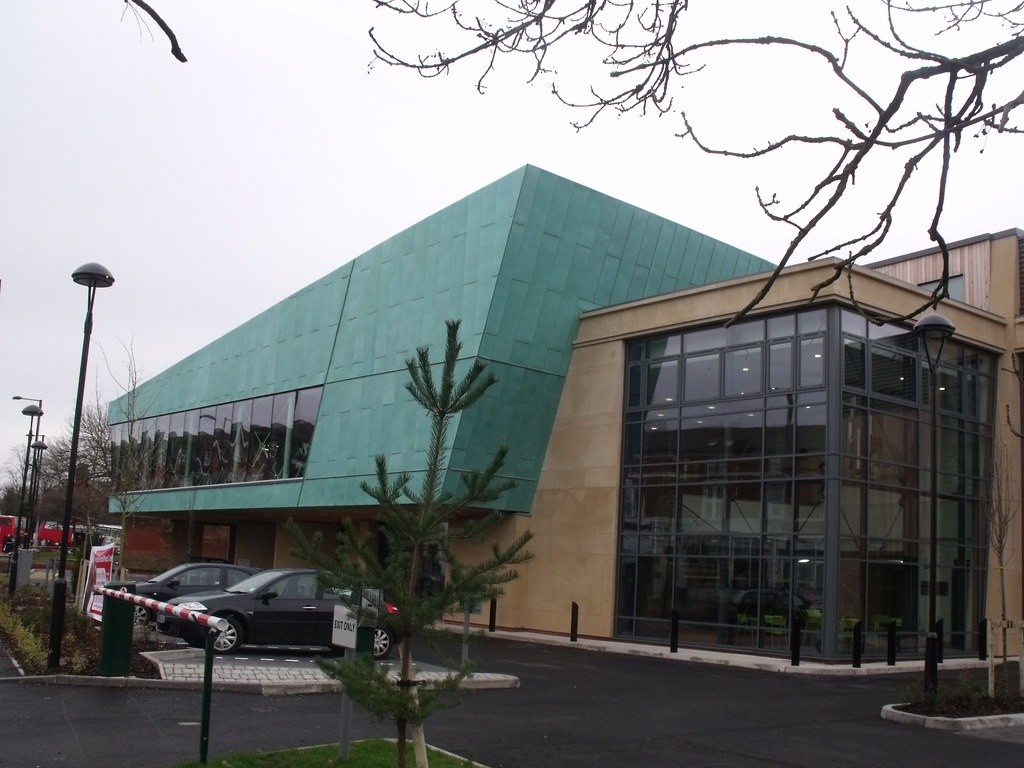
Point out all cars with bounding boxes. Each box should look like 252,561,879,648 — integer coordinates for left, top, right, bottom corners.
37,519,75,546
709,587,814,631
14,516,31,546
155,568,404,660
0,515,15,551
134,561,261,628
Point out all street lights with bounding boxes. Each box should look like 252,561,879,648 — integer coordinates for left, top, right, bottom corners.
9,395,49,596
913,311,956,701
48,261,115,668
